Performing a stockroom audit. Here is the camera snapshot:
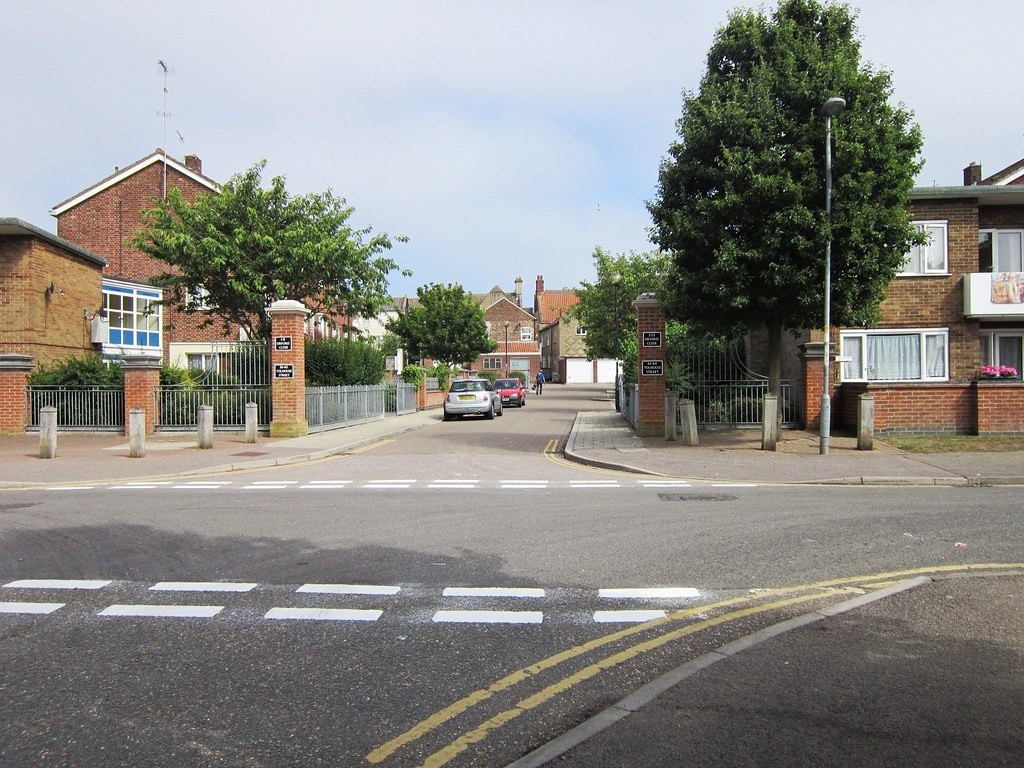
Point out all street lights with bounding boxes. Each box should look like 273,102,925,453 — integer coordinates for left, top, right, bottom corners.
818,96,848,454
609,270,622,413
502,320,509,380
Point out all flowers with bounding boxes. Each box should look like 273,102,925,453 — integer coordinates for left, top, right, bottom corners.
999,365,1017,376
981,365,998,376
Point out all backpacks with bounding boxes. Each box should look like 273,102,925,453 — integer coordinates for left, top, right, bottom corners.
536,376,542,384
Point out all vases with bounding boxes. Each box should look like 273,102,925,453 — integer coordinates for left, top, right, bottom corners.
981,375,1017,381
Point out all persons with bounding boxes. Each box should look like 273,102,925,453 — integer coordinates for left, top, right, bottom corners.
536,370,545,395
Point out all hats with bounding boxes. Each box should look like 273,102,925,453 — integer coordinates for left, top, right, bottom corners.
539,370,543,373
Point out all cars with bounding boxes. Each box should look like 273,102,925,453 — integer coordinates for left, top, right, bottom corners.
443,379,504,420
492,377,526,408
